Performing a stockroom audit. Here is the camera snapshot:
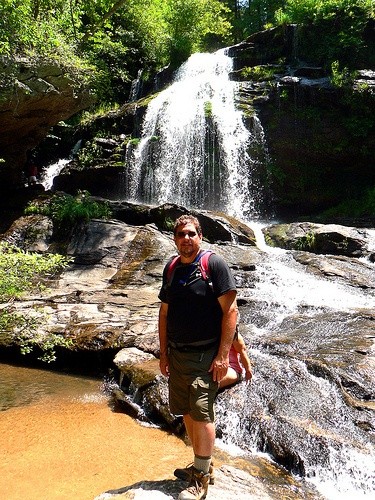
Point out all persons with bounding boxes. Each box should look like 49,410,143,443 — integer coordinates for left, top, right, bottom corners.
158,215,239,500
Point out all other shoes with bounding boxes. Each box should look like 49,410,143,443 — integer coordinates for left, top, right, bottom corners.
174,415,184,421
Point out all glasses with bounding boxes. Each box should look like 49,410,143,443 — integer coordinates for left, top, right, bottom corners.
176,231,197,237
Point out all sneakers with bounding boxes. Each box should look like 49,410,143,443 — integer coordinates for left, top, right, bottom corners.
178,468,210,500
174,462,215,485
